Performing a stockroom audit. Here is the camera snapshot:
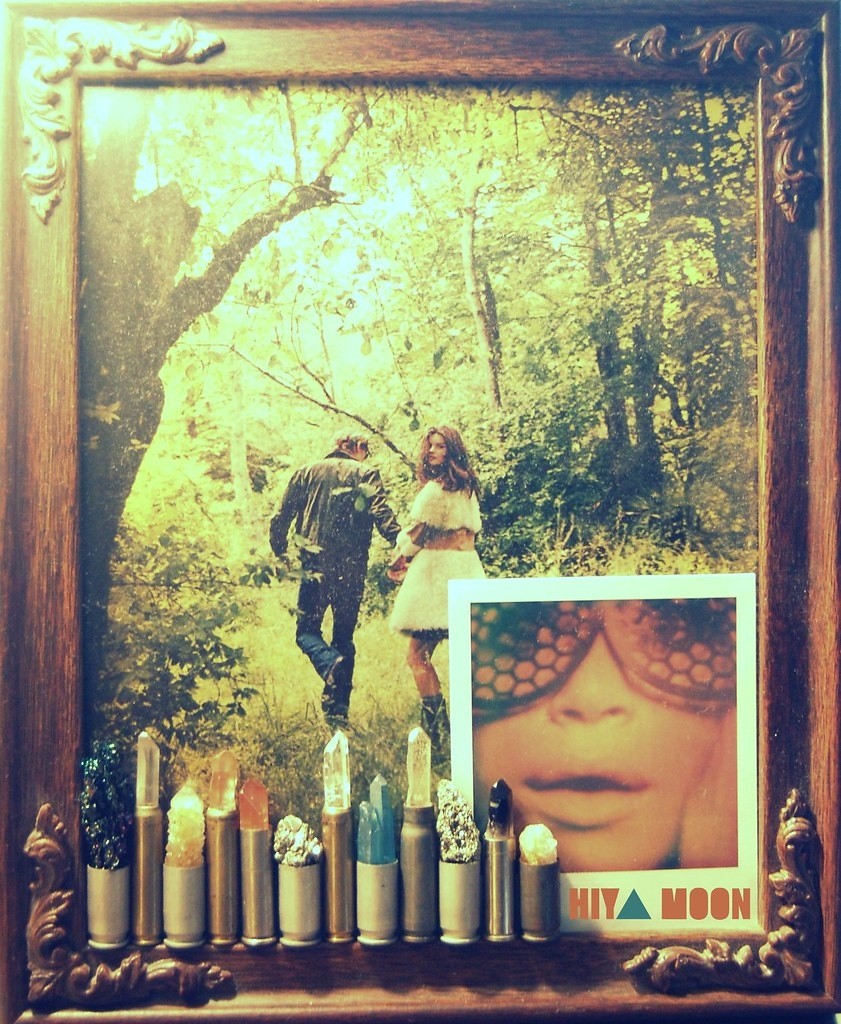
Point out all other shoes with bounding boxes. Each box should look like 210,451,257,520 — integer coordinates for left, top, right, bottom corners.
320,655,348,711
324,706,350,726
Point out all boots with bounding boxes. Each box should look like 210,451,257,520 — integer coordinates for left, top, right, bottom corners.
399,695,450,765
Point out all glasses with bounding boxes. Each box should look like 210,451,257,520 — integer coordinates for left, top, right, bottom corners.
364,448,370,460
471,597,736,711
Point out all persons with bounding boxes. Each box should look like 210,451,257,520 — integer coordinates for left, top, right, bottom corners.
388,425,489,768
270,429,414,729
472,599,738,869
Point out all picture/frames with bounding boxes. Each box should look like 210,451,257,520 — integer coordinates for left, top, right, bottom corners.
0,1,841,1024
